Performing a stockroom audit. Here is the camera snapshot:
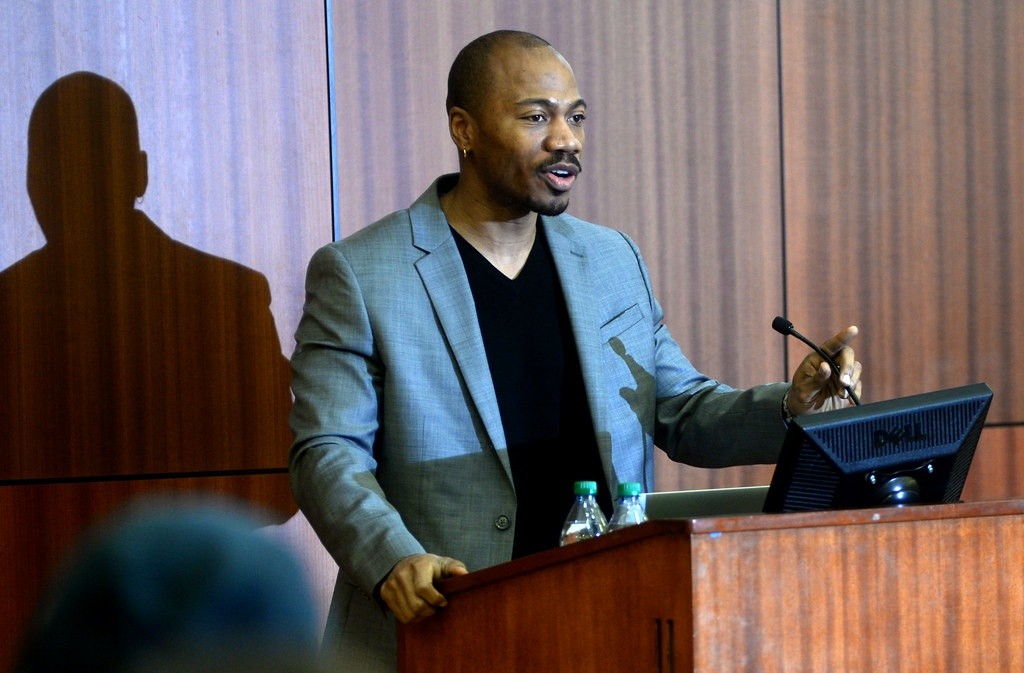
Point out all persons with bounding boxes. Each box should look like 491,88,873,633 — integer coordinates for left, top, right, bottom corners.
284,28,862,672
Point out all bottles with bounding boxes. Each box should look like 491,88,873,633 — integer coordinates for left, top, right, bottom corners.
605,482,649,533
559,481,609,547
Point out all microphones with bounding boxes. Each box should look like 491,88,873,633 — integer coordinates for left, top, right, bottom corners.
772,316,860,406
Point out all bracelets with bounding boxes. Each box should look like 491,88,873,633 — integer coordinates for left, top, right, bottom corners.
782,390,795,424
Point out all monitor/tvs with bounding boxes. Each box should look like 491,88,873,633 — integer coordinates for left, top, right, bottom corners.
761,381,993,515
